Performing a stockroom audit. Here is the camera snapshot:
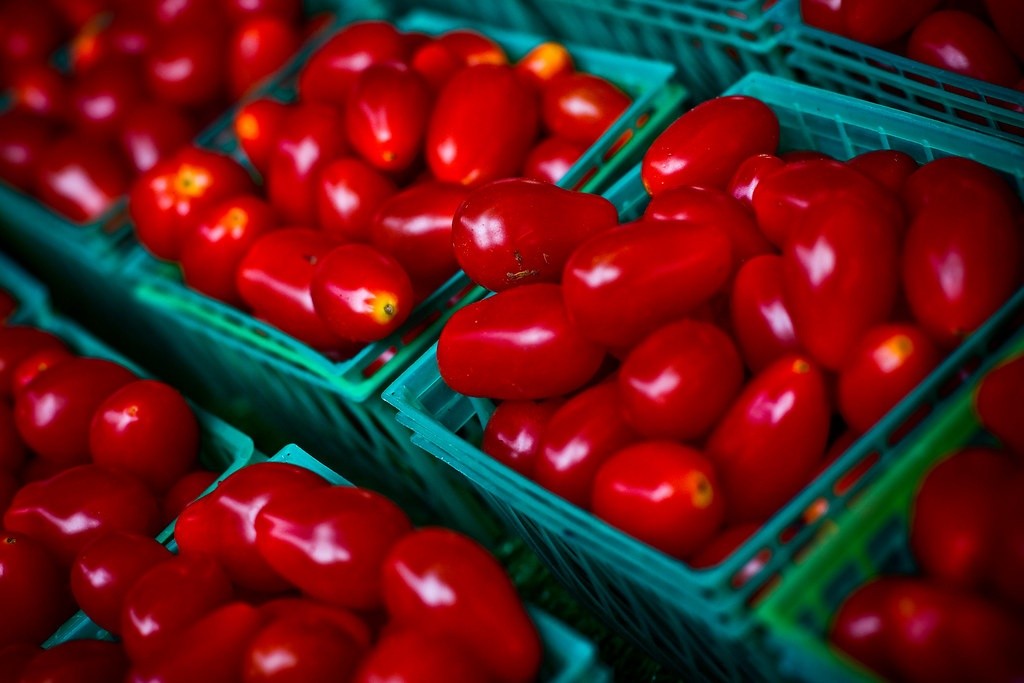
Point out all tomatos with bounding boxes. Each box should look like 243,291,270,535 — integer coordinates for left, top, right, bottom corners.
0,0,1024,683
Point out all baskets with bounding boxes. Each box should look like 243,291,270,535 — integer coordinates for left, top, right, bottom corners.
0,0,1024,683
